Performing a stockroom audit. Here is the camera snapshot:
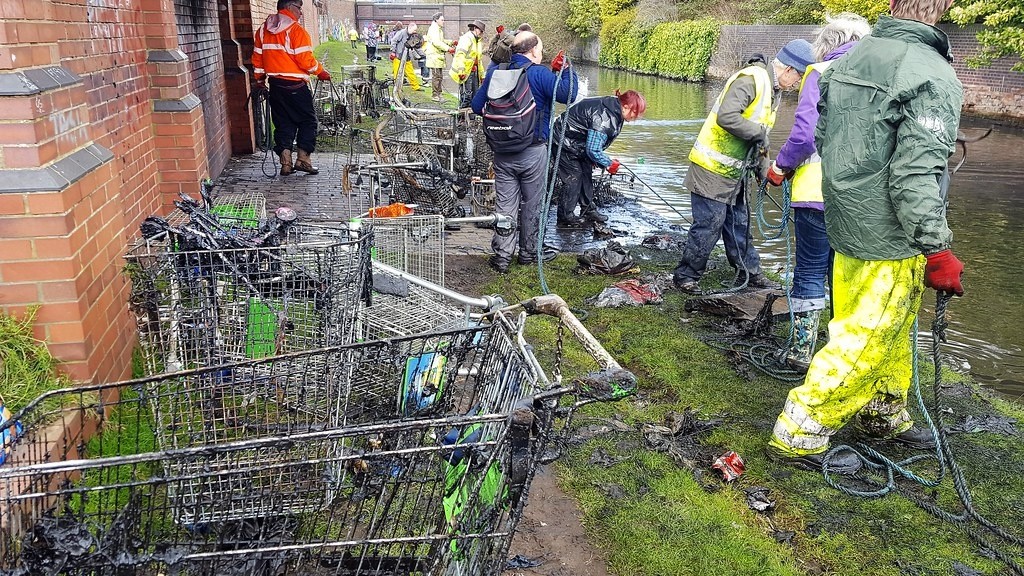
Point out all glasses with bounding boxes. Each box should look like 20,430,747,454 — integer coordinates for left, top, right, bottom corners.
290,3,302,11
796,70,803,83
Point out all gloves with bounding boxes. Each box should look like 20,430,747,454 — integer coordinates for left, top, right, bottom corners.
459,74,466,80
497,26,504,33
390,53,396,60
606,159,620,175
453,41,459,46
767,165,784,187
449,48,455,53
317,71,330,81
551,50,568,71
255,83,265,89
922,250,965,297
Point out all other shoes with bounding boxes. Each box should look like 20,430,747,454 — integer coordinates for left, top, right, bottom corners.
517,251,557,264
557,218,585,227
674,282,702,294
767,445,861,474
893,425,936,449
432,95,446,101
580,211,609,222
489,255,509,273
740,277,782,290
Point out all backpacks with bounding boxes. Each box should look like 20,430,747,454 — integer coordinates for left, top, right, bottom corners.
482,62,537,153
490,32,514,64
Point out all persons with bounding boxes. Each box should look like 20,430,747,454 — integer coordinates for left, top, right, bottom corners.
486,23,532,74
360,21,403,63
389,23,431,92
349,27,359,49
766,0,964,475
549,90,646,229
449,19,485,122
251,0,331,176
471,31,578,273
673,39,817,295
767,11,872,374
426,12,458,103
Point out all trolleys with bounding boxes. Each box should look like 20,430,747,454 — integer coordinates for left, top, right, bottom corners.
0,293,642,576
120,63,496,530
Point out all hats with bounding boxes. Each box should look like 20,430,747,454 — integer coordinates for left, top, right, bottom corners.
776,39,815,72
467,20,485,31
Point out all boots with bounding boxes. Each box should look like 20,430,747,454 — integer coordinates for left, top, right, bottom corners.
294,148,319,174
778,309,820,370
280,149,296,175
